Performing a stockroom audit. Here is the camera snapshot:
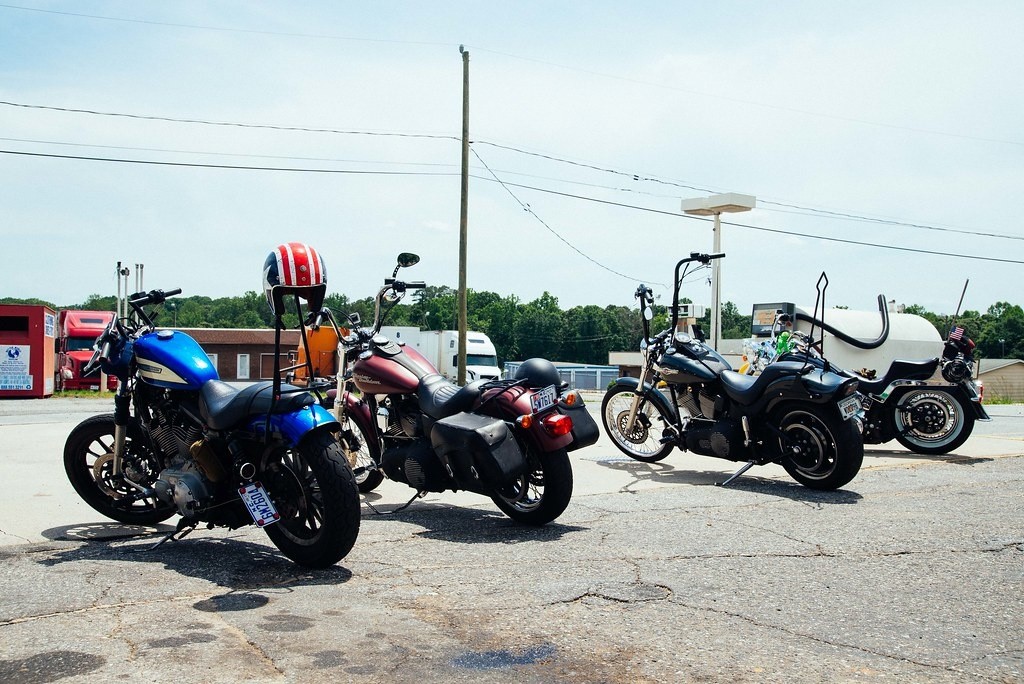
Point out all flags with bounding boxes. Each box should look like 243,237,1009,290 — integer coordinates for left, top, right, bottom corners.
949,325,964,340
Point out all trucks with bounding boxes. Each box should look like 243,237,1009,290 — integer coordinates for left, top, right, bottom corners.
54,311,122,397
349,325,503,391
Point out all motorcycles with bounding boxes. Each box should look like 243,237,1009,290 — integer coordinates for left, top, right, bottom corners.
296,251,601,528
739,309,991,457
600,252,866,493
63,287,364,571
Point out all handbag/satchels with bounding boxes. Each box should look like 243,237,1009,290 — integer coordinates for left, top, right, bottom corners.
295,324,350,379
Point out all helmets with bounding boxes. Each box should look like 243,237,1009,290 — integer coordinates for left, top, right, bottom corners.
515,358,561,393
262,243,327,317
